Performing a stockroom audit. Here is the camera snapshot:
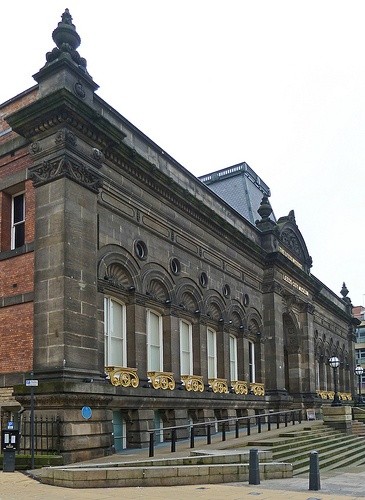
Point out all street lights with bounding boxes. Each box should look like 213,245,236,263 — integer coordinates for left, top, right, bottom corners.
354,366,365,407
328,355,342,407
